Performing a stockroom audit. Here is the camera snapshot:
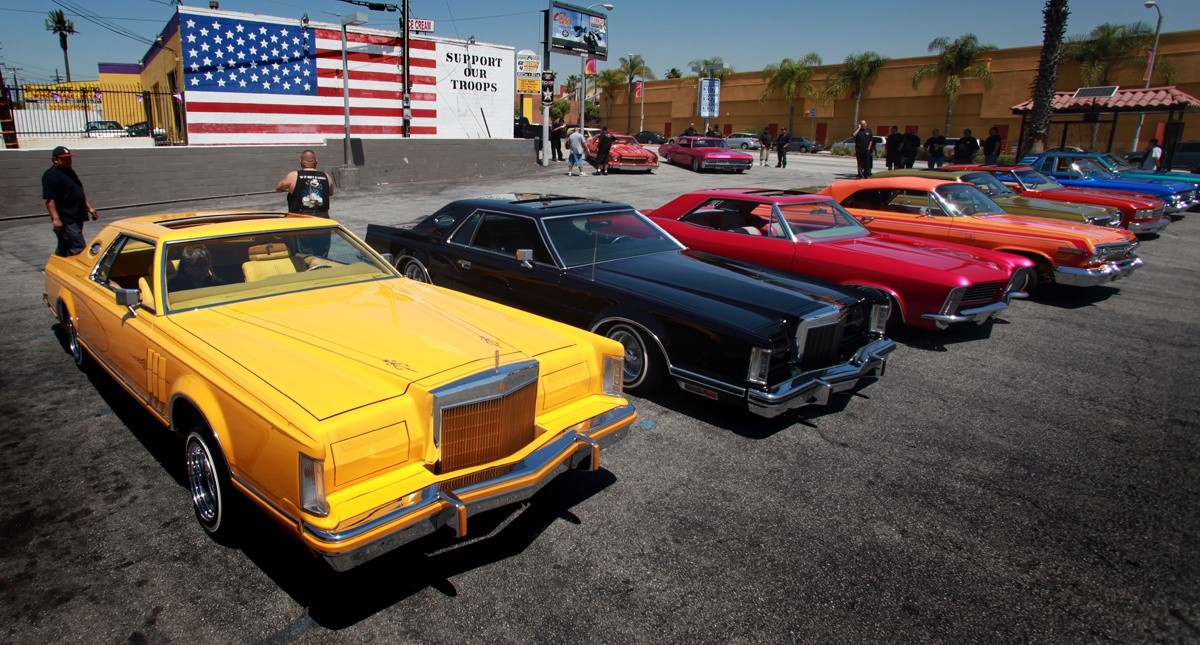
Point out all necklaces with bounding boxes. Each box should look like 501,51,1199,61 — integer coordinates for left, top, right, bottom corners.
592,126,618,175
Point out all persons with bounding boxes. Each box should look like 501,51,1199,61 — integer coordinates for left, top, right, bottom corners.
758,126,772,166
982,125,1003,167
175,241,230,290
683,122,698,136
1141,139,1162,170
566,127,590,177
865,136,878,177
40,146,100,257
704,127,716,147
923,128,947,168
775,127,791,169
852,120,872,179
551,116,566,162
897,127,920,169
884,125,902,171
274,149,335,260
952,128,980,166
713,124,723,147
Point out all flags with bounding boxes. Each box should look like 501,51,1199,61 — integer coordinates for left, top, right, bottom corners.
584,57,596,75
635,80,643,98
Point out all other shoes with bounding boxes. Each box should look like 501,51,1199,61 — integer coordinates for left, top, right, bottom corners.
568,171,572,177
592,171,600,175
775,165,781,168
783,165,785,168
765,164,769,166
760,163,763,166
552,158,557,162
559,158,566,162
579,172,588,176
602,170,607,175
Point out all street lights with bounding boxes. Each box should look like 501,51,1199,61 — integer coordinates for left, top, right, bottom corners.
340,12,368,168
627,52,634,134
1130,1,1165,152
580,4,615,137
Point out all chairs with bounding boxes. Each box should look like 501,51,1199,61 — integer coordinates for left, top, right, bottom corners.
240,243,311,282
729,226,763,236
139,258,182,303
761,222,786,237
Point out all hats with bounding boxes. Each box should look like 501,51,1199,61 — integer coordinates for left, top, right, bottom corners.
52,146,76,158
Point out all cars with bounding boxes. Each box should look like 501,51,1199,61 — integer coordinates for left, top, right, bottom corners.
917,138,981,161
772,137,822,153
582,133,660,173
575,127,603,143
669,133,707,146
41,141,1200,577
832,134,888,157
633,130,667,144
722,132,761,149
658,136,753,173
